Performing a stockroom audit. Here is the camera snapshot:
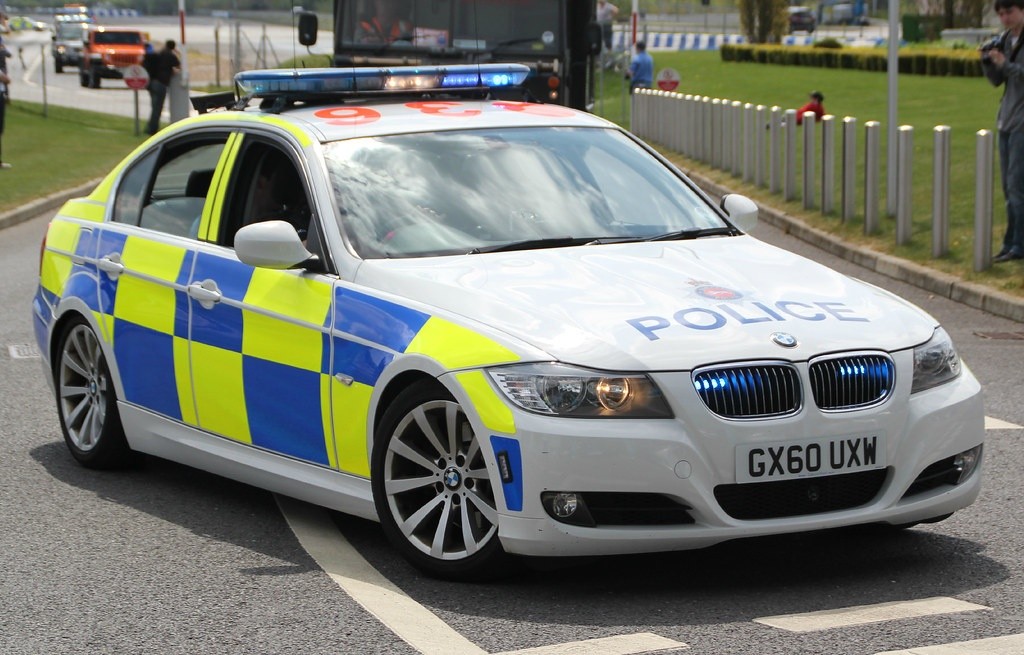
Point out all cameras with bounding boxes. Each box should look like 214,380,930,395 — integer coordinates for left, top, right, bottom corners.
981,38,1003,64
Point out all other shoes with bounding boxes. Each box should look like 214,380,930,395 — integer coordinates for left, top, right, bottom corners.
0,160,10,170
993,244,1023,265
145,124,159,135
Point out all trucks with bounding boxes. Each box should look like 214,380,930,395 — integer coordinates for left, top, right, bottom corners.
290,0,604,113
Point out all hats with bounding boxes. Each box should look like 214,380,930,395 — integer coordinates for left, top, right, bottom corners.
636,40,645,49
811,90,824,101
166,40,176,49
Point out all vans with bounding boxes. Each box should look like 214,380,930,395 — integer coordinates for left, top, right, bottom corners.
49,13,93,74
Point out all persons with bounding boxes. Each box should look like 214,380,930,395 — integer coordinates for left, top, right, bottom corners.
0,13,11,169
978,0,1024,263
766,90,826,131
139,36,181,136
272,167,312,248
627,42,654,92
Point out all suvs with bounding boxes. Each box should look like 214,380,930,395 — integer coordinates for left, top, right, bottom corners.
78,27,152,87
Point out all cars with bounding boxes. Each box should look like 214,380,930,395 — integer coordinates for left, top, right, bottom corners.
29,63,989,586
